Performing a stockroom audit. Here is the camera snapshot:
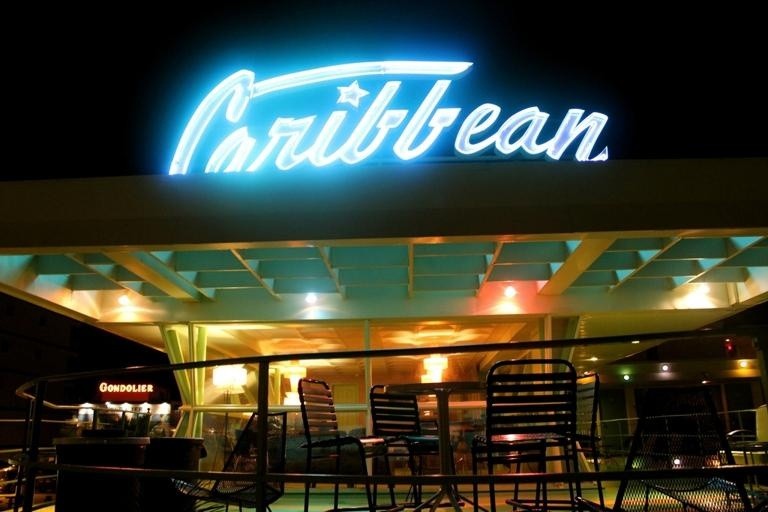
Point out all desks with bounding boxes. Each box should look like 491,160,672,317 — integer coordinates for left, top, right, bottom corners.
387,382,490,511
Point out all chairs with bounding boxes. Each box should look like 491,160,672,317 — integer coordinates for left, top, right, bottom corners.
724,404,767,500
298,377,396,511
471,359,584,512
610,385,761,512
369,382,461,512
172,413,288,512
510,371,604,512
